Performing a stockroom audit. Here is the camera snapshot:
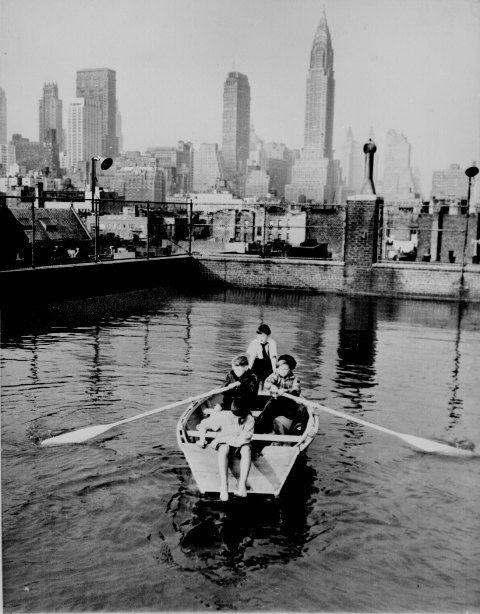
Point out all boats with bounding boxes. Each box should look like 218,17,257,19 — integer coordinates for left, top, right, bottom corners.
177,381,319,499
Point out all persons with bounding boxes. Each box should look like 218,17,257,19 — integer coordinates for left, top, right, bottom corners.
261,352,304,447
246,323,280,390
195,393,256,502
204,353,258,419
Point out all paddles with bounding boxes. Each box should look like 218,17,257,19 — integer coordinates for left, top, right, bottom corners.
40,381,240,445
283,394,478,455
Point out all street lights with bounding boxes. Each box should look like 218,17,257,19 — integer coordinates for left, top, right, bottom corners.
461,167,479,263
90,157,114,262
